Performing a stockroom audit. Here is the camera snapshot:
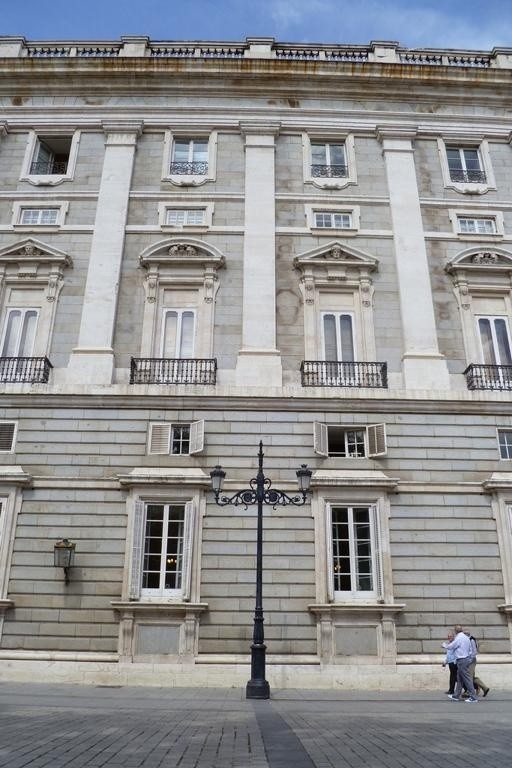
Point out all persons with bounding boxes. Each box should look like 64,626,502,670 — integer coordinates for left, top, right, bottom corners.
441,631,468,694
460,629,490,698
440,624,479,703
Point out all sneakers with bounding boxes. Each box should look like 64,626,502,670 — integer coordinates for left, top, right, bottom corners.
445,688,489,703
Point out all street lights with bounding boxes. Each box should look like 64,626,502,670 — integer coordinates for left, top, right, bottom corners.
210,440,314,697
